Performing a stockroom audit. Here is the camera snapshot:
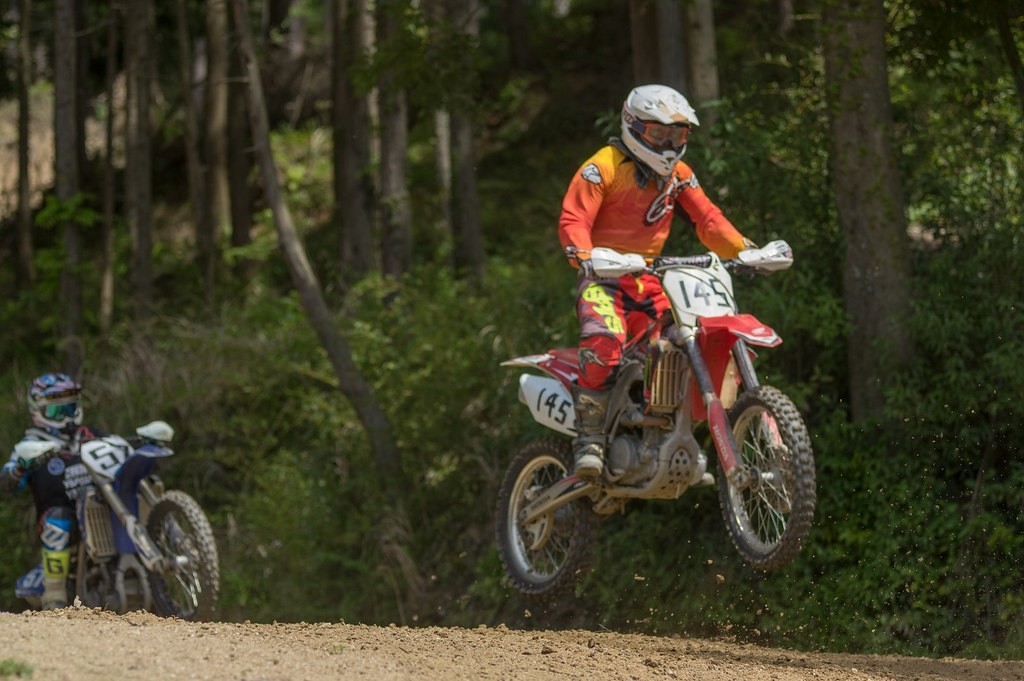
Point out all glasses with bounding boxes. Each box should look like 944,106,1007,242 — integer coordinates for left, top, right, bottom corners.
641,123,689,147
39,400,82,421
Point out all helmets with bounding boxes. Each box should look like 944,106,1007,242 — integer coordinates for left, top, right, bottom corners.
620,85,700,177
26,372,83,428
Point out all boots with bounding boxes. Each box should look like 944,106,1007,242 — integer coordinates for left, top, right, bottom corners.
40,546,70,611
570,378,614,477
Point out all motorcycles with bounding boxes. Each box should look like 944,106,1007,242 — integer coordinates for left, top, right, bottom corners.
491,246,816,608
12,418,223,627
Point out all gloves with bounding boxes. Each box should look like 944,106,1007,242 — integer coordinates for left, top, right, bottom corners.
17,450,54,472
139,434,168,447
729,259,772,285
576,257,608,281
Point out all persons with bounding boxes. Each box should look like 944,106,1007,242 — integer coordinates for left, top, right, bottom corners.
559,84,774,476
0,373,180,617
